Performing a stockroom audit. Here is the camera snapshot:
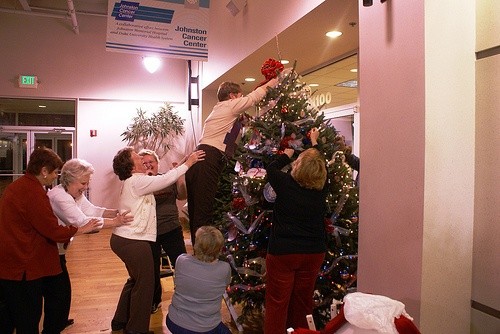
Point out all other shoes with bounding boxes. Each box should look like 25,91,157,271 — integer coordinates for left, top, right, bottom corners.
152,305,158,313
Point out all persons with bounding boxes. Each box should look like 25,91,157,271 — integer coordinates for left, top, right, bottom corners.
110,147,206,334
345,153,360,186
42,158,134,334
166,226,233,334
264,128,329,334
138,149,187,314
185,67,296,256
0,147,102,334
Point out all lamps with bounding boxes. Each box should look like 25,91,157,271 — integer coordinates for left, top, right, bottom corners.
141,56,164,74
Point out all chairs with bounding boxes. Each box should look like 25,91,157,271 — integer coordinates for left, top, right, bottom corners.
293,292,421,334
160,250,176,290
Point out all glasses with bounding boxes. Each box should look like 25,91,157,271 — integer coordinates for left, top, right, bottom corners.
236,94,243,97
